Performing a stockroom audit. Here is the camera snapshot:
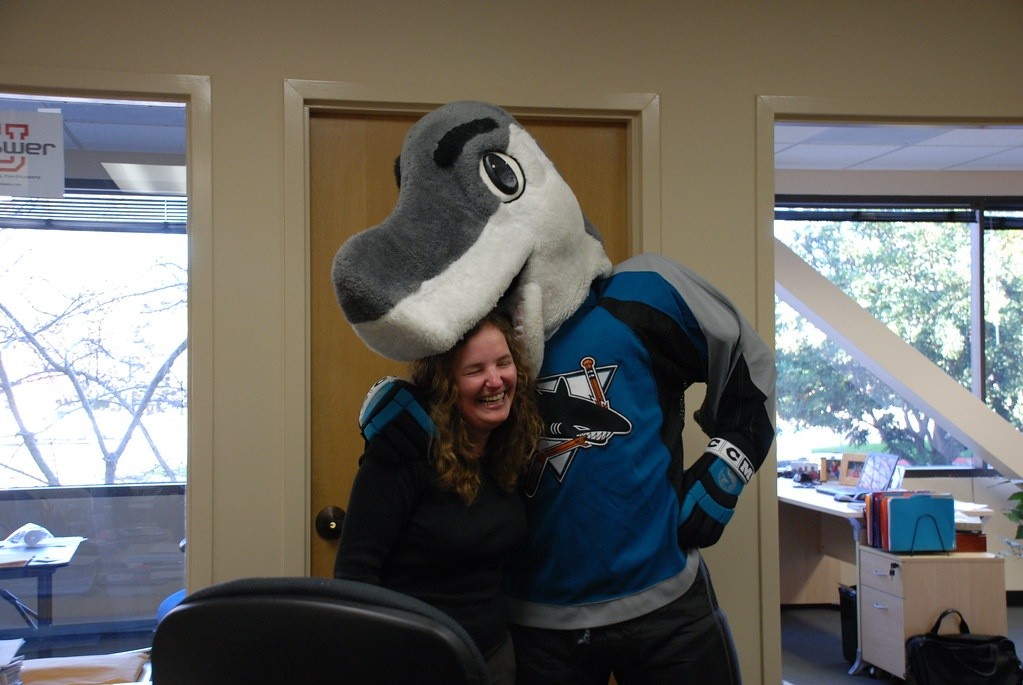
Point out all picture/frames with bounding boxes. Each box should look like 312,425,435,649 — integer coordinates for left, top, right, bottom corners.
819,456,841,483
839,452,867,487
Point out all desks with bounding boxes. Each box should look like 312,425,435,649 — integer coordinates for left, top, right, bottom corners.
776,477,987,674
0,535,88,659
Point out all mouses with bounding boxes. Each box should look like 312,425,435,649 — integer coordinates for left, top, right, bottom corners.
854,492,867,500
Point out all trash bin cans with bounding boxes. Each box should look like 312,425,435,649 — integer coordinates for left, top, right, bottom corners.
837,582,857,662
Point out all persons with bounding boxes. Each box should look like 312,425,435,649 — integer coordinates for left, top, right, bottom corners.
330,310,533,684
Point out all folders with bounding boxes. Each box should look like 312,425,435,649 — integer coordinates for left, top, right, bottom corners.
863,489,957,555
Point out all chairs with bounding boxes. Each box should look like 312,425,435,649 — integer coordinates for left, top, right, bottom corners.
152,576,492,685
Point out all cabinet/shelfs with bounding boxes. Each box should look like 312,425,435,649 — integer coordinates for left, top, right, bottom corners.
859,545,1008,681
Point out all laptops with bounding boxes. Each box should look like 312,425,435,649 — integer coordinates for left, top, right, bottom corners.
816,453,899,496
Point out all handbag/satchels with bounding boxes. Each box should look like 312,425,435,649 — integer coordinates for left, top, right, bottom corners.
906,608,1023,685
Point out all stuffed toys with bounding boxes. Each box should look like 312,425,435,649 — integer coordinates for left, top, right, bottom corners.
332,101,780,684
